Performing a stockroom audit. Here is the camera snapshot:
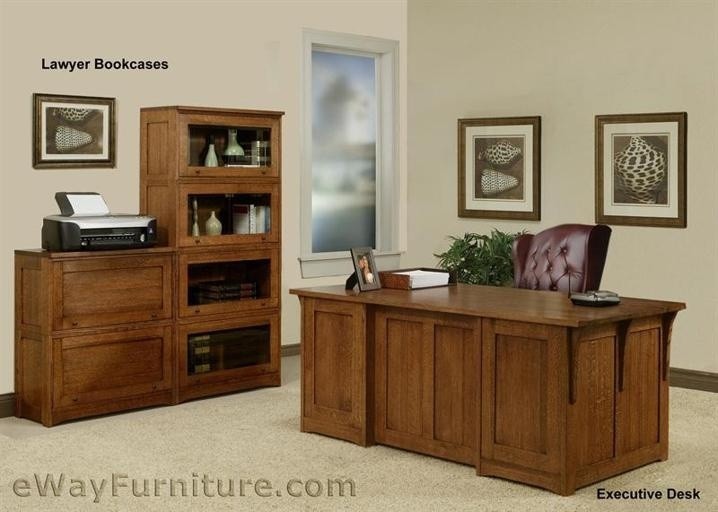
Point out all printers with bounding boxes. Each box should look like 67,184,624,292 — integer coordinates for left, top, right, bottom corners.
40,192,158,252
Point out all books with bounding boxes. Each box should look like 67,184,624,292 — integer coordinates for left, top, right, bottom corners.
186,333,212,374
197,279,258,306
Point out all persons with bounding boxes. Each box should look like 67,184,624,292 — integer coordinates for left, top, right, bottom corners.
361,255,376,284
356,255,371,284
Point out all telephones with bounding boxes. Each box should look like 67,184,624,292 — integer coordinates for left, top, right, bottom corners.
568,273,620,307
